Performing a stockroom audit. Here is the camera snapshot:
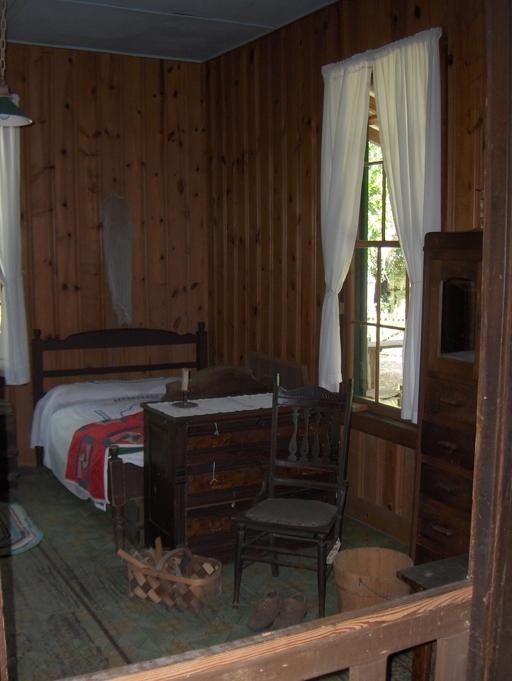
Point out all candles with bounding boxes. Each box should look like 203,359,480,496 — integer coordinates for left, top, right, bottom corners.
180,367,189,391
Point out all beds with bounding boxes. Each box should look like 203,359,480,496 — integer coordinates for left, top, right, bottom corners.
31,320,305,554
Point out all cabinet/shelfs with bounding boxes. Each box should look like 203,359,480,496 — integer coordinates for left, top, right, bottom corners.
140,388,336,551
407,228,482,564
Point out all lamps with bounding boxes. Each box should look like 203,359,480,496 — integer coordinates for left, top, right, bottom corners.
0,1,33,127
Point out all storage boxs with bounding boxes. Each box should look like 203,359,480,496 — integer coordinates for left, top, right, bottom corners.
128,549,222,608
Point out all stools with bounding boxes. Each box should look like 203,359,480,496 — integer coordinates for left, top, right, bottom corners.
394,559,469,680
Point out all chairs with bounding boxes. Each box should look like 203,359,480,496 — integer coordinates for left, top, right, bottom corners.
232,371,354,619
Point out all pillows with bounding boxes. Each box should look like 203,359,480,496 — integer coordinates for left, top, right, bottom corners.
245,349,301,389
167,369,238,397
